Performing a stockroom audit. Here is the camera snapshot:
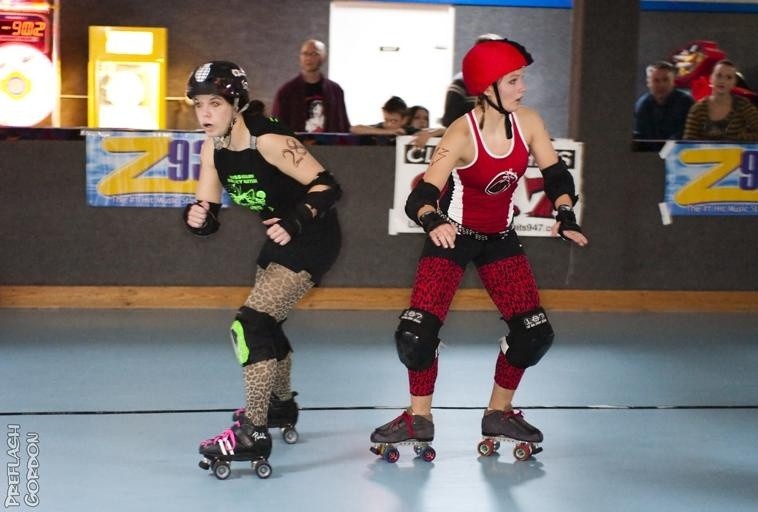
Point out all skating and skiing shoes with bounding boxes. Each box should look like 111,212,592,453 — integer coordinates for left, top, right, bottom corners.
199,421,272,479
232,390,299,444
370,411,436,462
478,409,543,460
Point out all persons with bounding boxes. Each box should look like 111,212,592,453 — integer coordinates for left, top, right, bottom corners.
634,59,696,139
350,95,445,151
182,59,344,458
437,32,504,127
684,59,758,141
406,105,430,130
271,39,350,132
370,39,590,445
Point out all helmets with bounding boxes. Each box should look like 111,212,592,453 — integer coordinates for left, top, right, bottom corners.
463,39,533,95
185,61,249,112
